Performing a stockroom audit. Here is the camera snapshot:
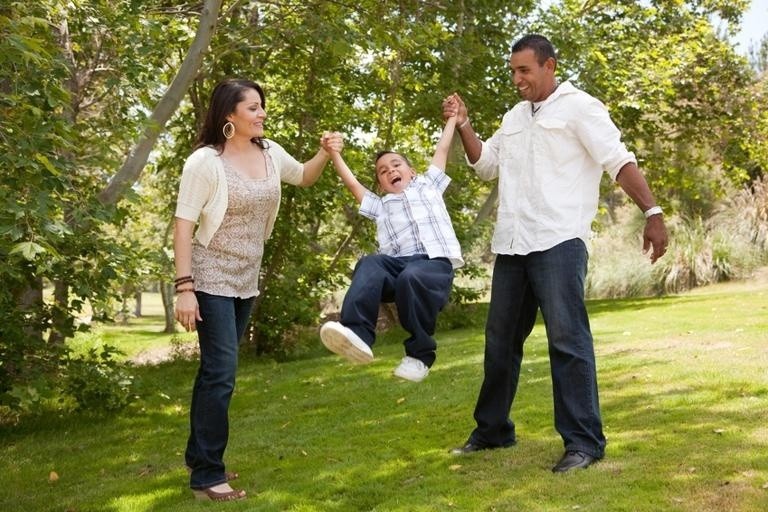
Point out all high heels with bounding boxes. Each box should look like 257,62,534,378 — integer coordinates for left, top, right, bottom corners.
186,465,240,480
192,488,247,502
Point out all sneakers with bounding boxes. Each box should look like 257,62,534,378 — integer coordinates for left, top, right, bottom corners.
394,355,430,382
320,322,373,365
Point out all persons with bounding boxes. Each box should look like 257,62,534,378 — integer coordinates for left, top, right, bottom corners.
319,95,465,383
441,35,670,473
175,77,343,502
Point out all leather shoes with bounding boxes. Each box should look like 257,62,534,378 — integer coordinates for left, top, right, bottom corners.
550,450,597,475
449,440,488,455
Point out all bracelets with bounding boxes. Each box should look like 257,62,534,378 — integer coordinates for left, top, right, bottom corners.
454,117,471,128
643,206,665,218
174,277,196,294
